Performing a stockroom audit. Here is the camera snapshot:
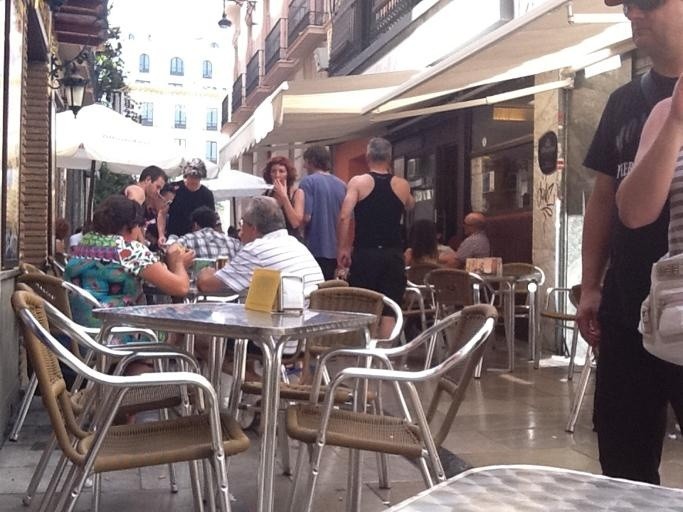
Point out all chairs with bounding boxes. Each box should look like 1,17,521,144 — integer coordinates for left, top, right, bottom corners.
423,268,501,378
243,279,350,383
502,263,545,360
11,289,250,508
567,285,599,432
228,286,404,490
403,277,435,356
17,283,202,506
286,303,498,512
532,288,585,379
10,254,104,442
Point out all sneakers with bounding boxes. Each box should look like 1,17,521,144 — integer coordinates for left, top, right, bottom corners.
238,382,262,430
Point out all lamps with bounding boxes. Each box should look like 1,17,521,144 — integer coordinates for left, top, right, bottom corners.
47,62,90,119
46,37,92,119
218,0,244,29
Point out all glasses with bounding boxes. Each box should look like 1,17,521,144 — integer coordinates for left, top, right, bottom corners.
623,0,661,18
239,217,255,230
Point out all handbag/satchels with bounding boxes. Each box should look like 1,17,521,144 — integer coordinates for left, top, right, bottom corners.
637,251,683,366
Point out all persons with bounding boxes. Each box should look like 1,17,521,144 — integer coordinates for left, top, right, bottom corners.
338,137,415,346
574,0,683,486
55,160,245,267
300,145,354,279
195,195,326,427
614,69,683,374
442,213,492,268
436,225,454,261
261,155,305,242
64,196,197,373
403,221,445,342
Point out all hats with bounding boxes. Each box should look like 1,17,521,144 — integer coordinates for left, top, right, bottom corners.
183,159,206,178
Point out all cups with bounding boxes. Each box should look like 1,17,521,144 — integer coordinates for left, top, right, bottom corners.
194,258,215,274
218,255,229,268
